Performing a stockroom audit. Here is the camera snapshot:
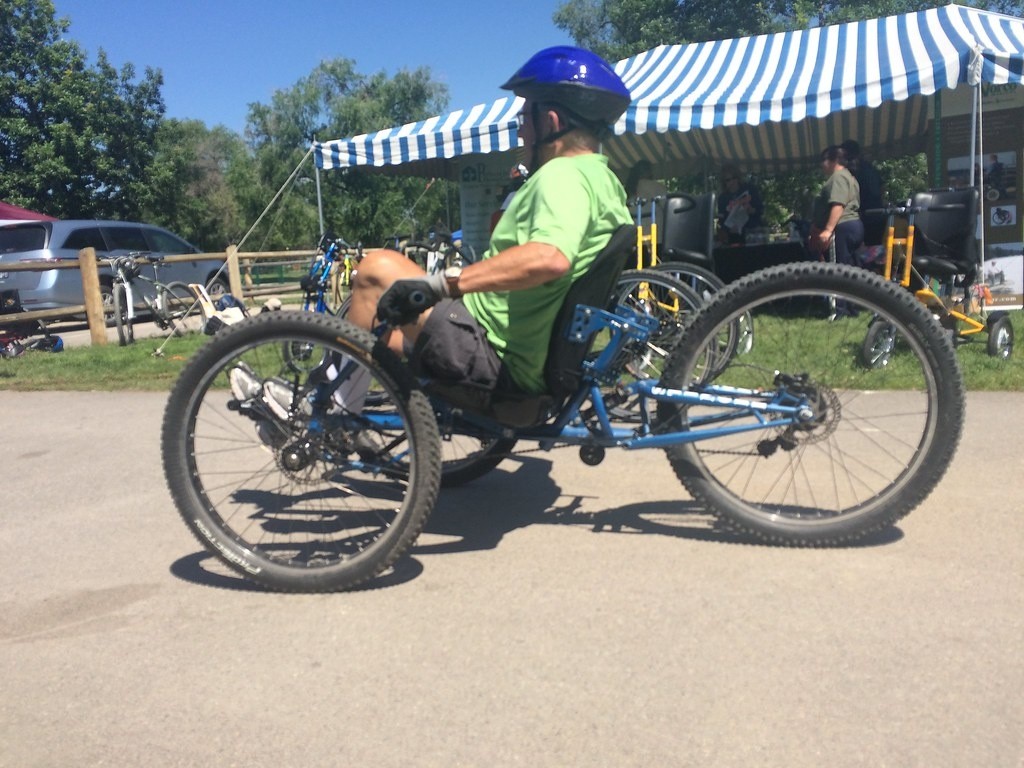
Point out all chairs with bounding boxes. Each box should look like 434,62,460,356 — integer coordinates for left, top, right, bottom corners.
629,191,716,299
0,286,59,350
897,189,980,288
403,208,639,431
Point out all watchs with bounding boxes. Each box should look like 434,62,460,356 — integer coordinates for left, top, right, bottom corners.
444,267,462,299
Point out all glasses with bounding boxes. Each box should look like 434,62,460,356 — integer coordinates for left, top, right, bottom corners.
516,110,546,127
822,157,830,162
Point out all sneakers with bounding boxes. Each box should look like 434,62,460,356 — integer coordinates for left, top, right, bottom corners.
228,357,362,453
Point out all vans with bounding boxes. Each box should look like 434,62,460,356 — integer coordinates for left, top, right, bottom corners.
0,220,231,327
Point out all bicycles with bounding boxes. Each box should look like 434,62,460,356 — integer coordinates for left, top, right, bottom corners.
97,251,207,346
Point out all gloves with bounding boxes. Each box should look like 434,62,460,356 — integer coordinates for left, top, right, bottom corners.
375,269,452,321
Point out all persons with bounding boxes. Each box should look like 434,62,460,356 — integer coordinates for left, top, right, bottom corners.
625,160,666,246
717,164,764,245
840,140,886,246
985,155,1004,184
229,45,632,455
819,146,864,323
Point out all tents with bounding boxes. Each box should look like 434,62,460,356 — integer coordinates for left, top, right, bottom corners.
312,4,1024,287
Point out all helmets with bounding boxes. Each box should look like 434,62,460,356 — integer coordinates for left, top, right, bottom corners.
499,46,631,126
